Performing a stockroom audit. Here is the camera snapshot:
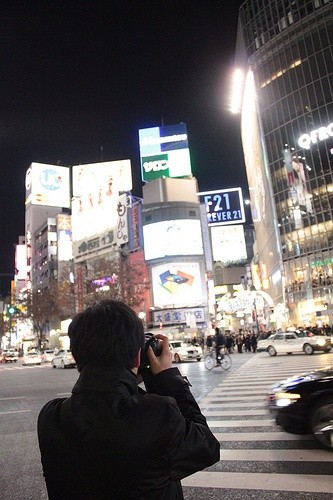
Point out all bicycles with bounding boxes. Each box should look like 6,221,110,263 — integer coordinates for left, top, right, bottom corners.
204,346,232,370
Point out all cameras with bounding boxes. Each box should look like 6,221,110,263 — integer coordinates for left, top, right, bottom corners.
140,333,160,364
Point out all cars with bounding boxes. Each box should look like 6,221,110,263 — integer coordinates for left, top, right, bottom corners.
41,350,56,361
257,329,333,356
168,341,203,363
52,349,78,369
267,366,333,450
21,353,41,366
1,349,21,357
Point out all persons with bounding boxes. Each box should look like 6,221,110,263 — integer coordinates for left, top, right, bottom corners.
213,327,224,364
37,299,220,500
187,324,333,354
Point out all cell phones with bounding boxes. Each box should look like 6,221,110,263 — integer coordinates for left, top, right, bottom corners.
165,274,188,284
